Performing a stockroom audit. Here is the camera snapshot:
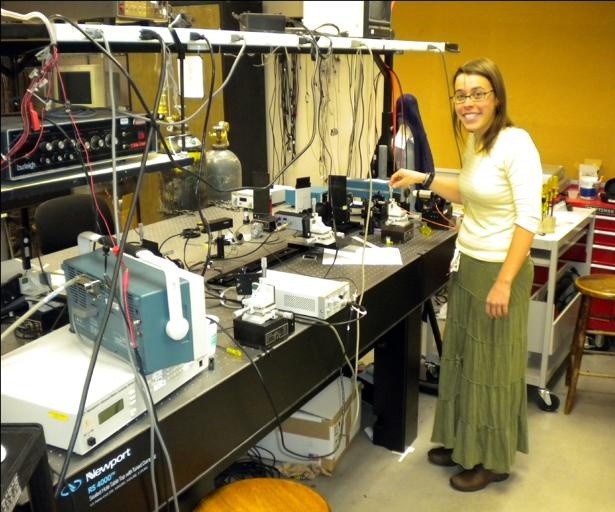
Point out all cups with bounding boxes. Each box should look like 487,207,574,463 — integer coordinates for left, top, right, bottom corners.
579,176,600,200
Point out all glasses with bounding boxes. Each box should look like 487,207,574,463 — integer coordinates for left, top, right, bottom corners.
450,89,495,104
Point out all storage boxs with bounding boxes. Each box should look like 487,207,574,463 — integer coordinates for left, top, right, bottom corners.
245,376,361,463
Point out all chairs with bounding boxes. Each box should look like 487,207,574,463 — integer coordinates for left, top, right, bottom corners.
33,195,114,255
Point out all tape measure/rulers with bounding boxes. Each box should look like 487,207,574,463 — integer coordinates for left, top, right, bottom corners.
351,236,380,248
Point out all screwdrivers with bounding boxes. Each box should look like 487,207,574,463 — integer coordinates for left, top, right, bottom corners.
542,175,558,217
217,345,242,357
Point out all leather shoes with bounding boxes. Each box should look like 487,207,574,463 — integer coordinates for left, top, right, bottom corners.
429,447,512,492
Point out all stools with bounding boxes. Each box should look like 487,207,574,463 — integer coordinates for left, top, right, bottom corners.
190,477,331,512
564,274,615,415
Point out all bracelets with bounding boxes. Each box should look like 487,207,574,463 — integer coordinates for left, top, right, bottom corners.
421,172,434,190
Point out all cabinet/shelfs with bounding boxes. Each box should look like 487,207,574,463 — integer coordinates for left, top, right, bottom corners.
526,208,597,411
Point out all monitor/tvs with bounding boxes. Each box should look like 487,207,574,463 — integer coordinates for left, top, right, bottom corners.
51,64,106,108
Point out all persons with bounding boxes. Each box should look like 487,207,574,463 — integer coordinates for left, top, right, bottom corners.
388,54,544,495
